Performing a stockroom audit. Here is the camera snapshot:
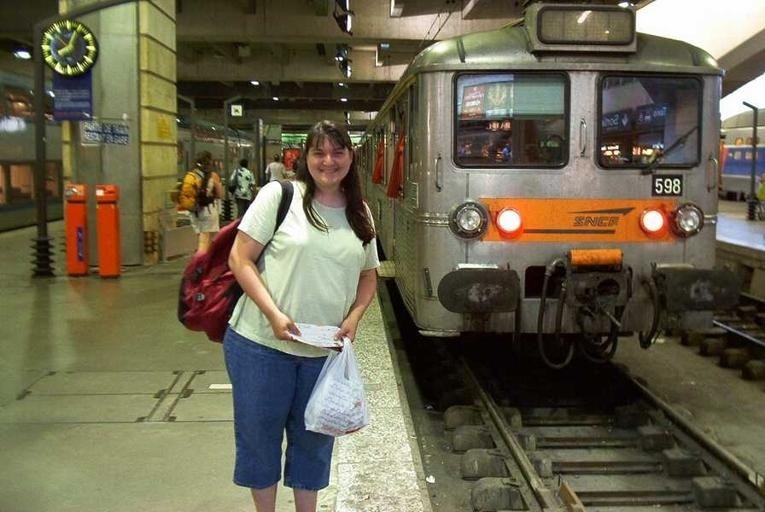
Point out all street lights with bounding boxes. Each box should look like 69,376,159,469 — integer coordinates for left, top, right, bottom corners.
221,95,244,220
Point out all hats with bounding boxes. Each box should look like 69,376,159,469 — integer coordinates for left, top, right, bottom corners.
193,150,213,162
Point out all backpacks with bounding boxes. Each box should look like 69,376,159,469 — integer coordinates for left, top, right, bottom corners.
179,168,213,213
176,179,294,343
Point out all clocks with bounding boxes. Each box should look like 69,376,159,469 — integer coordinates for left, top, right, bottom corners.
41,18,98,79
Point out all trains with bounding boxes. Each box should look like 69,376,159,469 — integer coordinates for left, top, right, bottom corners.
718,109,765,202
355,1,740,368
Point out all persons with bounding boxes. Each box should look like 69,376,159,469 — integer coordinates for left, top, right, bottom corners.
188,151,225,253
223,121,381,512
228,158,259,219
265,154,289,184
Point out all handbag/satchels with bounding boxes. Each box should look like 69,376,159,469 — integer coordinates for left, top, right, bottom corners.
265,171,271,182
227,172,237,194
